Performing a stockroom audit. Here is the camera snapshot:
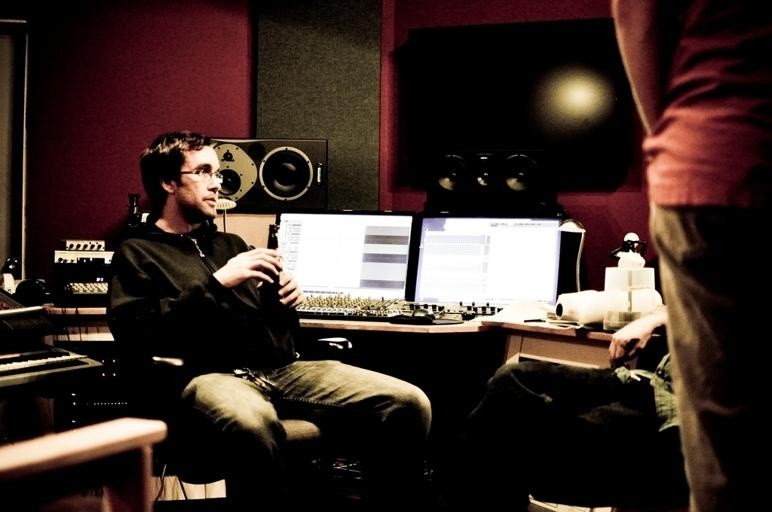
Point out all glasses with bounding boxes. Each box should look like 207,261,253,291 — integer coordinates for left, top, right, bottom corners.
179,168,224,184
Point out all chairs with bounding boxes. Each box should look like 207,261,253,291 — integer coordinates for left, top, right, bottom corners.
151,338,353,511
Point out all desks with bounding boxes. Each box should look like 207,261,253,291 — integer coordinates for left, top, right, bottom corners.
500,319,666,375
41,304,497,338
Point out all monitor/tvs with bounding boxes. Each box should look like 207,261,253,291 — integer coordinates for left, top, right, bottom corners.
276,213,416,301
141,211,279,291
410,213,562,316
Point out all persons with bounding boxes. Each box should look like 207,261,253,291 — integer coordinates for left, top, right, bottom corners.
107,131,434,512
613,0,772,512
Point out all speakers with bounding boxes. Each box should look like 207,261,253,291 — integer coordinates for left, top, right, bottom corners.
422,142,557,219
205,139,329,213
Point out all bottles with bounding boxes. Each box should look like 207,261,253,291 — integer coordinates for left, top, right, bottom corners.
313,160,326,210
259,221,284,316
118,192,143,243
